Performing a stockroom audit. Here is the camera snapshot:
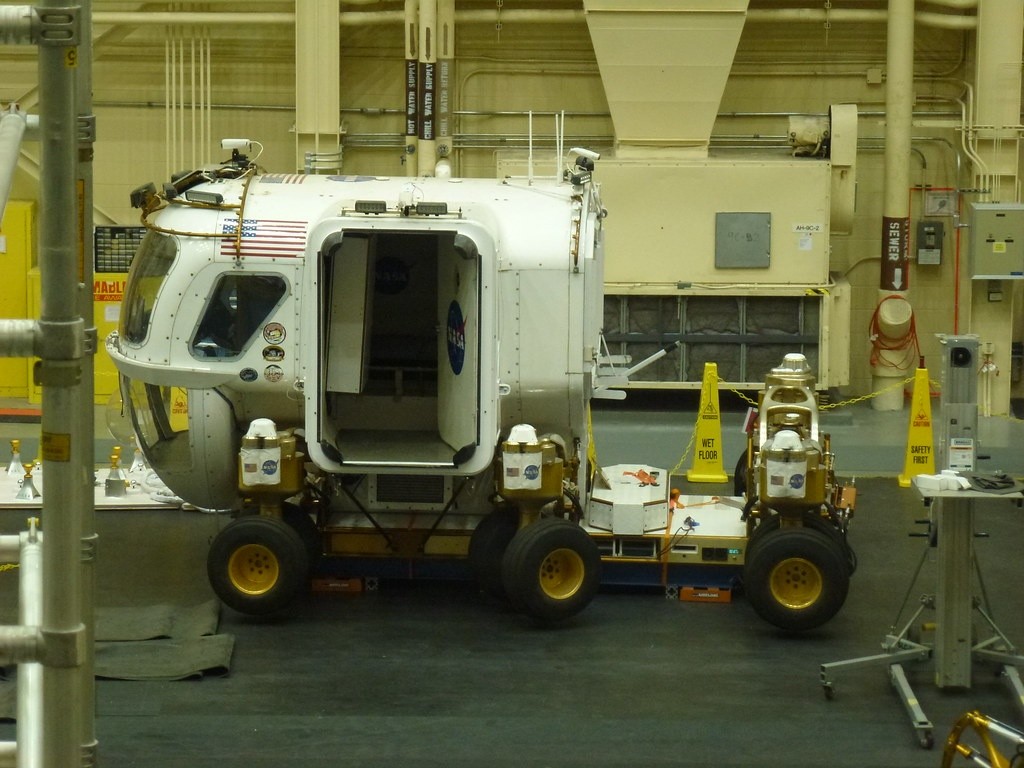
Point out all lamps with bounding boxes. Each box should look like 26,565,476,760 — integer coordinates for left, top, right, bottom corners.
572,170,591,185
166,169,223,204
342,200,462,218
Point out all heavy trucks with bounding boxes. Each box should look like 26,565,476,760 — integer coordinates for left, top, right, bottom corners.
104,139,858,634
